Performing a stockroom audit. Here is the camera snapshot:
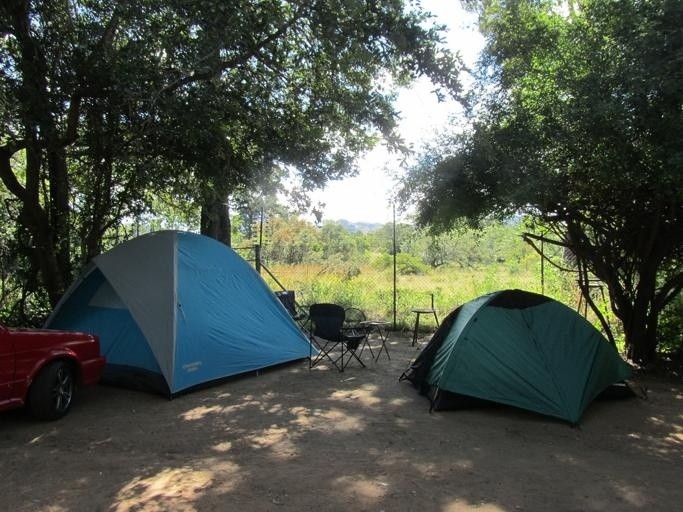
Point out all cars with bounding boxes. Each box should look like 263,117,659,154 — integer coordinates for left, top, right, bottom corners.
0,318,108,425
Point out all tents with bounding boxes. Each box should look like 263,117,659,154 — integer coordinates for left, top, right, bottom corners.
399,288,648,430
38,228,321,402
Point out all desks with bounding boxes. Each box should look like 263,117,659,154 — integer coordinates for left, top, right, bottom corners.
359,320,392,362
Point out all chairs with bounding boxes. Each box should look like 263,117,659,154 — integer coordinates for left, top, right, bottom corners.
412,294,440,347
275,291,305,329
341,308,375,369
575,276,610,329
308,304,366,372
